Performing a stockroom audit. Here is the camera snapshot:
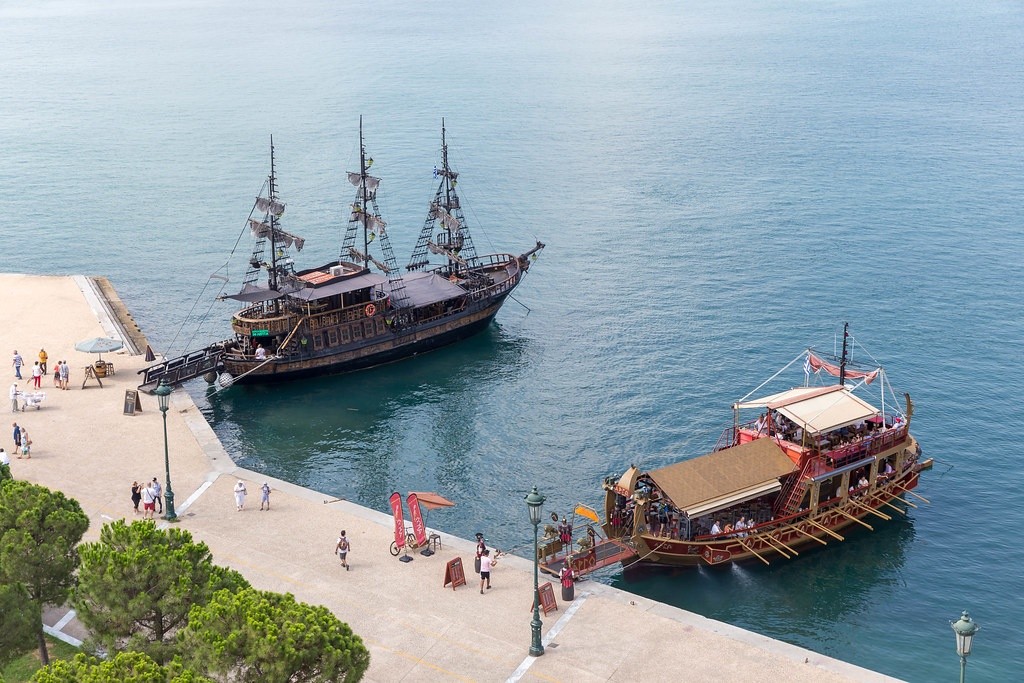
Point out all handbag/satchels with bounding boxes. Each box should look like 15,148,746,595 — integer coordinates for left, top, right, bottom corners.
26,440,32,445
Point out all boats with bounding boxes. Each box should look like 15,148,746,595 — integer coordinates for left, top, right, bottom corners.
600,322,934,567
218,112,547,388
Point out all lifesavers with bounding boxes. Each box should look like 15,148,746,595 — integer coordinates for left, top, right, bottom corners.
365,304,375,318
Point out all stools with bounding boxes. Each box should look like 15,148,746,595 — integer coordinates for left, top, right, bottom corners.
105,363,115,376
85,366,93,379
428,534,441,553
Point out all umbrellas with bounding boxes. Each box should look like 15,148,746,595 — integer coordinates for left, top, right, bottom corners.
145,344,157,371
409,491,455,528
72,337,124,363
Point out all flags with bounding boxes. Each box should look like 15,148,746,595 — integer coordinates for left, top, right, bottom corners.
365,157,374,170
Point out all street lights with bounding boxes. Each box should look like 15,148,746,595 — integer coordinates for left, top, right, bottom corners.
948,608,983,683
523,484,547,657
153,376,177,522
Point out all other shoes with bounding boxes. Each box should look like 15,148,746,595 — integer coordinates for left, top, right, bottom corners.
480,591,483,594
267,509,269,511
260,508,263,510
487,586,491,589
158,511,162,514
346,565,349,571
341,564,345,567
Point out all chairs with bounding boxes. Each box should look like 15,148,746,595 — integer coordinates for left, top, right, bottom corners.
786,430,797,442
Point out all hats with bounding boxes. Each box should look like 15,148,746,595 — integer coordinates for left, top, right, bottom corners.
238,480,243,483
896,418,901,422
152,478,156,481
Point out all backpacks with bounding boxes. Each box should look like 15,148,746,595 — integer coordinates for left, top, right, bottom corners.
339,537,347,550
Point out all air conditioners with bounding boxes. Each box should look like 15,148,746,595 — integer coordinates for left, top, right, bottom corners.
330,265,343,277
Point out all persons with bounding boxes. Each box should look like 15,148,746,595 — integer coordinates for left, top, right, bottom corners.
141,477,162,520
335,530,350,570
909,442,922,461
260,482,271,511
558,518,572,544
0,422,32,466
255,344,265,360
754,408,800,441
234,480,246,511
480,550,497,594
822,417,906,449
711,516,758,540
836,457,892,497
610,485,673,536
9,347,69,411
131,481,142,513
